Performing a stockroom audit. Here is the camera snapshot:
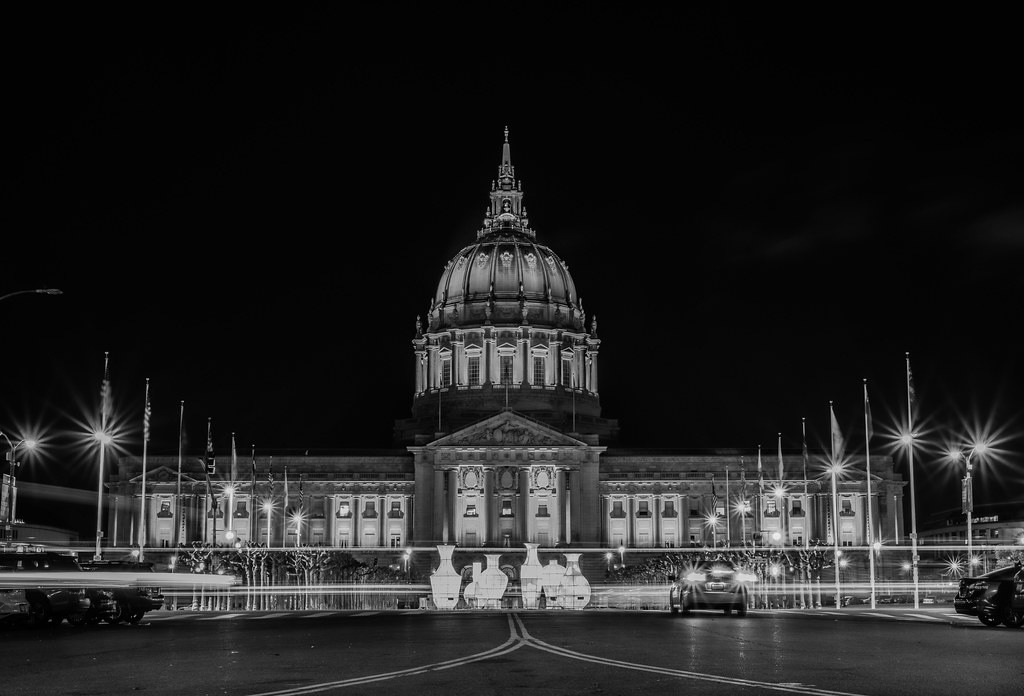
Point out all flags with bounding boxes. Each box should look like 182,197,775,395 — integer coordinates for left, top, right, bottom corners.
231,438,237,485
269,460,274,496
144,395,151,439
712,483,718,517
284,470,288,509
831,407,843,458
181,426,187,449
252,451,256,490
299,478,303,510
101,367,112,413
206,433,214,476
740,467,747,501
908,366,915,403
865,397,873,443
778,437,783,480
802,435,808,462
758,451,764,489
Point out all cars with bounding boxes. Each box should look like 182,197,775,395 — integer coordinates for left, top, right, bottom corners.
668,560,748,617
1012,563,1024,627
953,562,1021,628
0,542,91,635
77,558,166,625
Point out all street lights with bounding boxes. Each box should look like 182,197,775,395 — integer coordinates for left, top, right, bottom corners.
774,485,788,609
946,441,990,578
606,552,613,573
407,548,412,581
828,464,844,608
619,546,624,567
293,515,302,546
709,515,721,551
263,502,273,548
226,486,234,532
900,434,921,609
94,432,112,561
0,431,38,544
738,503,749,547
